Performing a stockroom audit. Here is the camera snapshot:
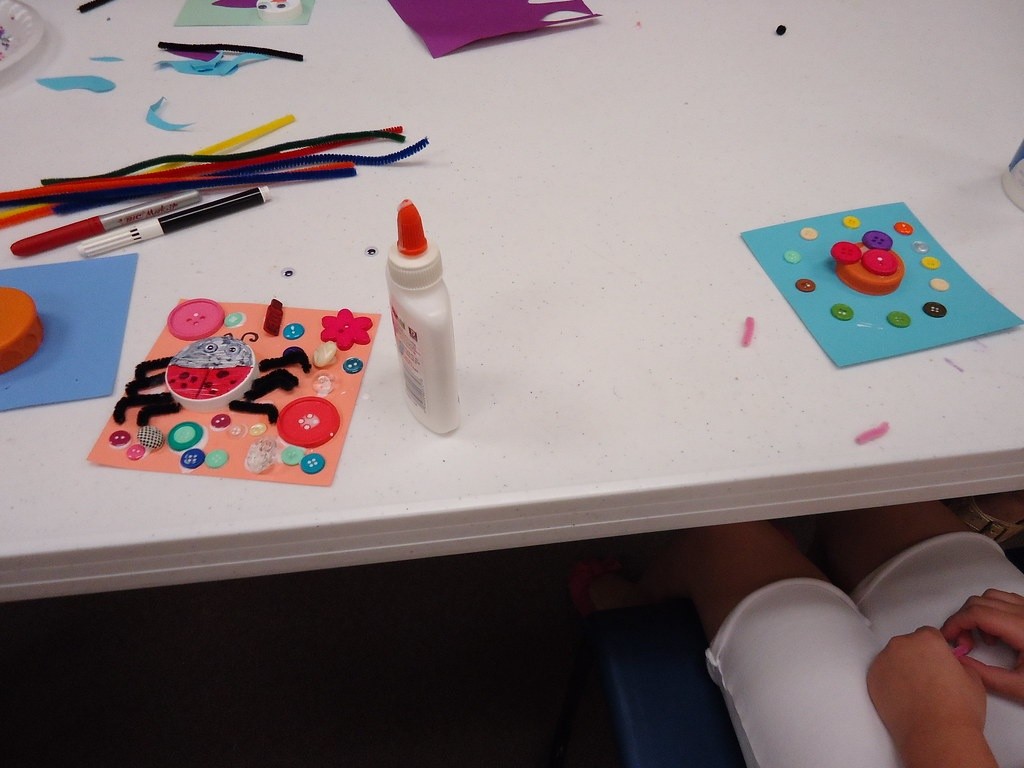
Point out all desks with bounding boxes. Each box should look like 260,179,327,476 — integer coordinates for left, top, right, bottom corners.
0,0,1024,606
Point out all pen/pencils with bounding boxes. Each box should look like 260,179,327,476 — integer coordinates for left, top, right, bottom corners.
76,184,274,258
10,189,202,257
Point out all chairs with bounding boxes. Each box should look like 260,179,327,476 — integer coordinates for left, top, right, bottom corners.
548,547,1024,768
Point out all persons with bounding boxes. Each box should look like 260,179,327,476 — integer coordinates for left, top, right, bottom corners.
568,491,1024,768
